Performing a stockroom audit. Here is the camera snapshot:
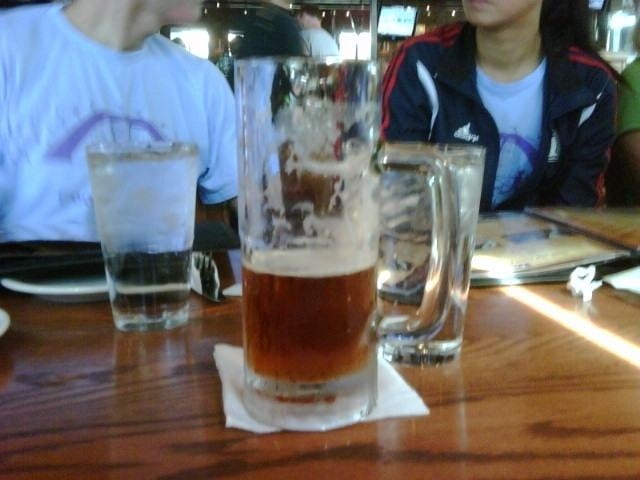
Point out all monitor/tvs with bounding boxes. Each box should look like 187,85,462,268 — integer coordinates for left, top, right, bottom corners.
377,2,419,40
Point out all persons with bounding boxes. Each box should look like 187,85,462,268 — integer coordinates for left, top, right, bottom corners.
615,57,639,204
0,1,239,253
336,1,625,219
206,0,337,124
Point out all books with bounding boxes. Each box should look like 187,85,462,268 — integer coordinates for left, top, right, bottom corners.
371,204,640,307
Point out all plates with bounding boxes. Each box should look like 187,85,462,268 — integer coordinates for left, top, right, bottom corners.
2,276,107,304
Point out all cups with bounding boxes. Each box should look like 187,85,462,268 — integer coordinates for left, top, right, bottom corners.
87,144,198,332
232,55,460,434
375,141,486,371
